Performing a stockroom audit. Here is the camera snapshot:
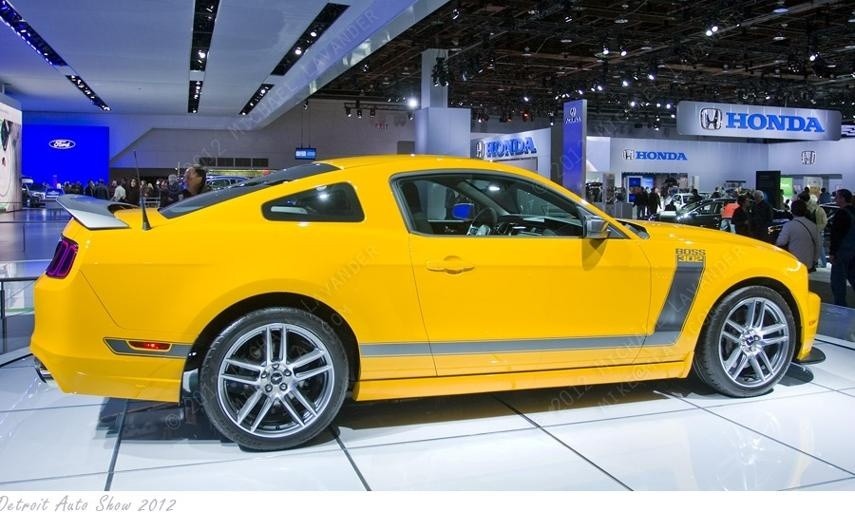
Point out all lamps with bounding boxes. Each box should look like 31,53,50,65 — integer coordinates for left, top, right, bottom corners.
343,0,855,132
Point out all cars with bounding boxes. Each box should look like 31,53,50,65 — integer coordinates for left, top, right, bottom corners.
28,153,822,453
178,174,251,193
639,198,793,250
666,192,694,212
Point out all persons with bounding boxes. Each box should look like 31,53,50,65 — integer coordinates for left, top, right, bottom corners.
691,189,702,201
588,184,626,204
629,185,663,219
711,186,855,308
56,174,179,211
664,201,677,211
172,162,214,205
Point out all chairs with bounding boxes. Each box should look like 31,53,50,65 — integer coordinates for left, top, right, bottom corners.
400,182,433,233
